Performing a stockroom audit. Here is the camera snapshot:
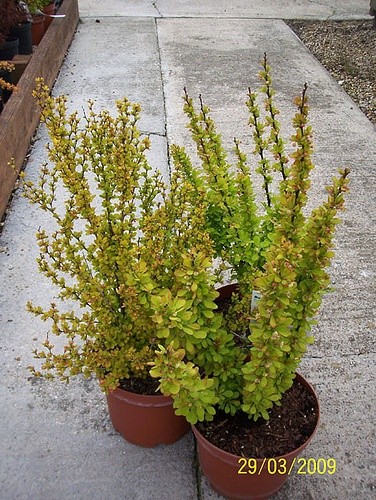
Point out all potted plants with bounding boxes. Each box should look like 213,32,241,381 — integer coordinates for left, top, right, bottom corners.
129,152,350,497
169,49,315,348
8,70,198,450
